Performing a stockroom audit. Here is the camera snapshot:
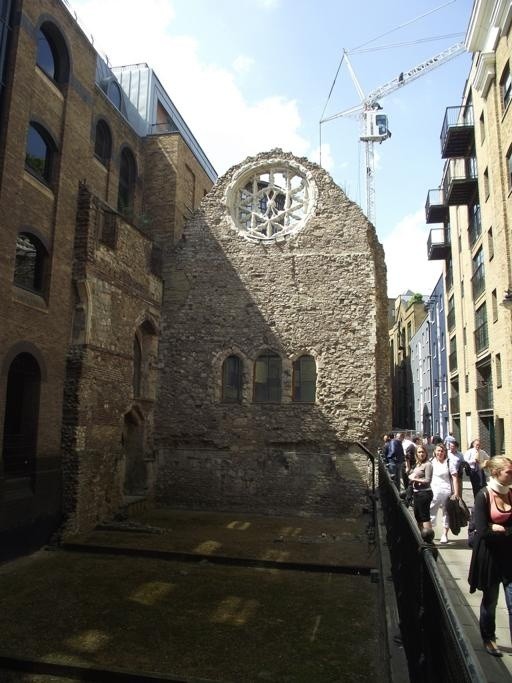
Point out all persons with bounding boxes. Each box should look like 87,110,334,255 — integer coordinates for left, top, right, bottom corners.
378,428,512,657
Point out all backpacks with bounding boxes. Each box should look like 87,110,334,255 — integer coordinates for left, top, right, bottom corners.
405,444,417,470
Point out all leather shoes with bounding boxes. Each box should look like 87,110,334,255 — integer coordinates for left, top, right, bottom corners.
485,642,501,656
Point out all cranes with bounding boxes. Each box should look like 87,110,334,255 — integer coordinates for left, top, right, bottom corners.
318,0,467,225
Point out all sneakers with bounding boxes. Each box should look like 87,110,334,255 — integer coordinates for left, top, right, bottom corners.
440,534,448,543
